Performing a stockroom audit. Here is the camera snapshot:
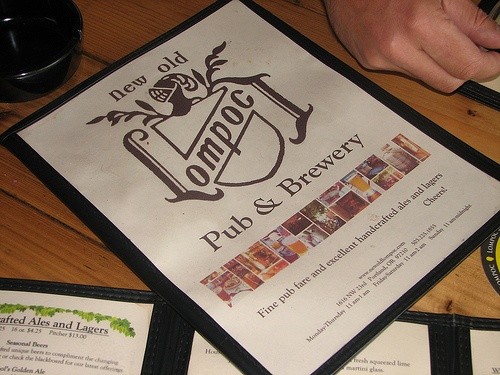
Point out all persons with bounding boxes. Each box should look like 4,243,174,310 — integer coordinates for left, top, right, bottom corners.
324,0,500,95
